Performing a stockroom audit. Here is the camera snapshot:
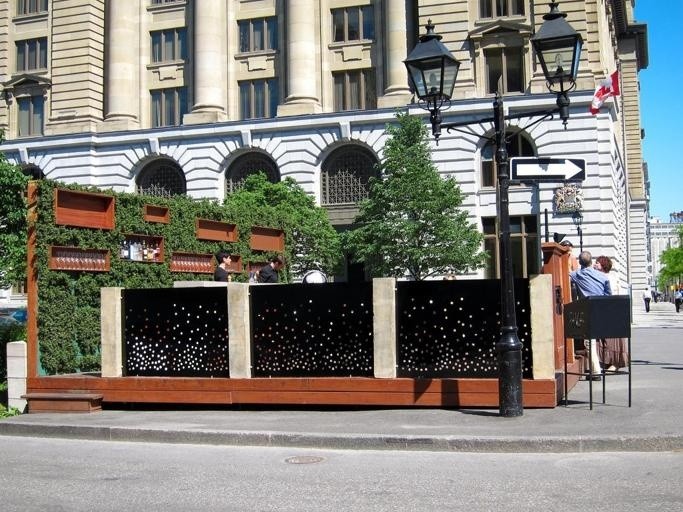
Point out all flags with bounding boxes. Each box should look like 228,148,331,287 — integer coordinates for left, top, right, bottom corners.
590,69,621,116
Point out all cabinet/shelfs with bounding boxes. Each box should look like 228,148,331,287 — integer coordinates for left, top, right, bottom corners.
47,187,284,276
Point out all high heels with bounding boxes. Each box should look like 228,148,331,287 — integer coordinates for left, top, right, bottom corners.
603,364,618,375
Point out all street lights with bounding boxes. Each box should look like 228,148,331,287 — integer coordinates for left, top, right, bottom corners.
402,0,584,418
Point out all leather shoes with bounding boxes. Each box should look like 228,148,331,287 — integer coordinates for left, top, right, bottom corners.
585,370,601,381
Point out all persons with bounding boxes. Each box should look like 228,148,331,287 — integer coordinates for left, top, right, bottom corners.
653,289,658,302
675,288,683,312
642,287,652,312
569,251,627,381
214,249,231,282
258,255,286,283
